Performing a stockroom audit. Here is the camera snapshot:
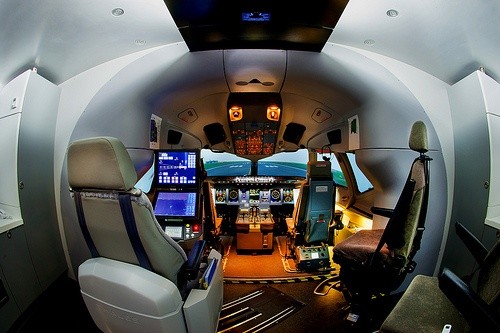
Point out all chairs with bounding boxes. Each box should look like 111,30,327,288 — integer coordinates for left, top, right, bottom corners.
200,157,224,257
332,120,434,318
66,137,224,333
374,219,500,333
285,161,344,250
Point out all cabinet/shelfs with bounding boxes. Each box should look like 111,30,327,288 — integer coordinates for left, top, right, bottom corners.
0,68,68,333
441,69,499,283
